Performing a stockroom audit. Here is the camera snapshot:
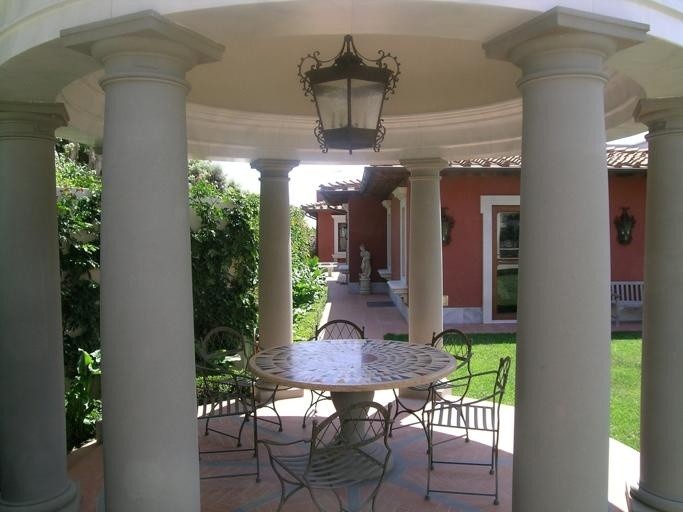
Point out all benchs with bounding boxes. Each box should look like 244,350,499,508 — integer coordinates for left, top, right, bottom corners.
317,262,337,276
611,281,644,327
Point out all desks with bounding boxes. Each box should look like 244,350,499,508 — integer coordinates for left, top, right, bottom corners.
248,339,457,482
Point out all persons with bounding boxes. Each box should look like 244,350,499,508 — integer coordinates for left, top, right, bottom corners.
359,245,372,277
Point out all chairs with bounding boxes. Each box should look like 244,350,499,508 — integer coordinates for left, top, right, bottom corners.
422,355,511,506
301,319,366,429
389,328,474,454
195,326,282,446
266,399,393,512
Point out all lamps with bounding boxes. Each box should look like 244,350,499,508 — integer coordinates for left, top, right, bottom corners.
298,34,402,154
441,206,456,246
614,207,637,245
